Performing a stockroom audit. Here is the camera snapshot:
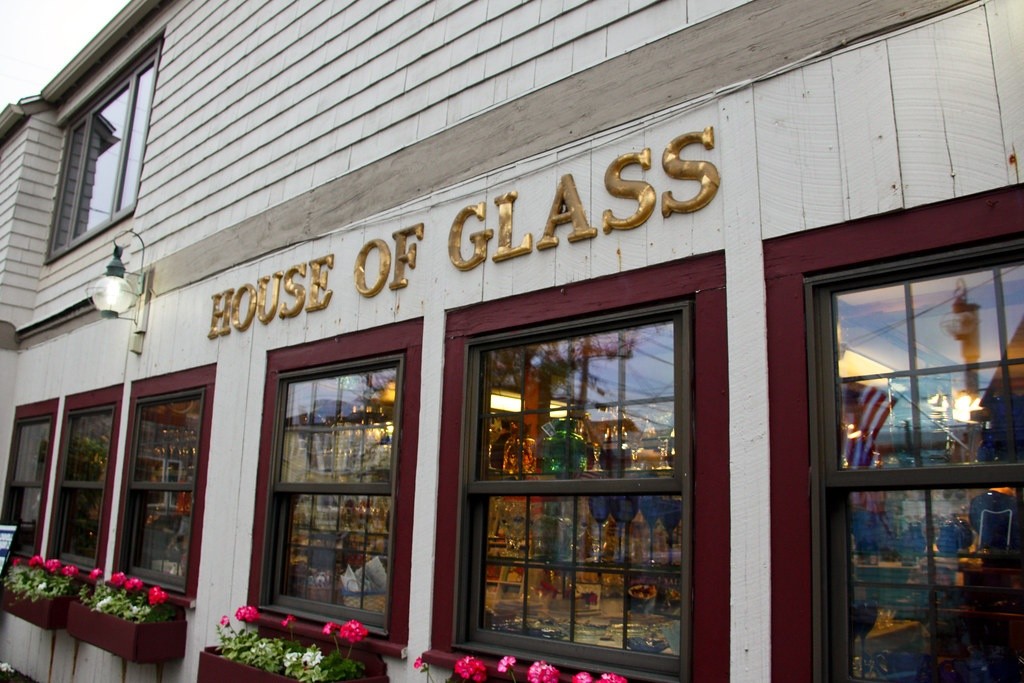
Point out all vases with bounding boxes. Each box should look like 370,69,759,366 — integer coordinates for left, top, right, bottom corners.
197,646,389,683
0,680,14,683
443,673,512,683
66,600,187,662
0,581,75,630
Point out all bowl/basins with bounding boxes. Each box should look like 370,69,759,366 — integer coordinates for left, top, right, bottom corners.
483,614,680,657
628,585,657,614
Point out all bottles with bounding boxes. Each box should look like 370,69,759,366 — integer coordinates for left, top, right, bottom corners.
505,405,678,475
308,404,393,484
148,428,196,483
852,508,974,567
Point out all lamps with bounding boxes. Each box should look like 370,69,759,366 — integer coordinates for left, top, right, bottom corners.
85,228,146,326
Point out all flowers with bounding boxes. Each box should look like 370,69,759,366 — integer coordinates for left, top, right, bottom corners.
215,605,369,683
414,655,627,683
0,662,15,680
3,555,77,607
78,568,177,623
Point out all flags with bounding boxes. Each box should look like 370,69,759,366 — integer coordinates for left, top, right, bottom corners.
835,380,897,469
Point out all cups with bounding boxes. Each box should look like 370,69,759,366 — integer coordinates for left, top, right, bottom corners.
875,649,988,683
